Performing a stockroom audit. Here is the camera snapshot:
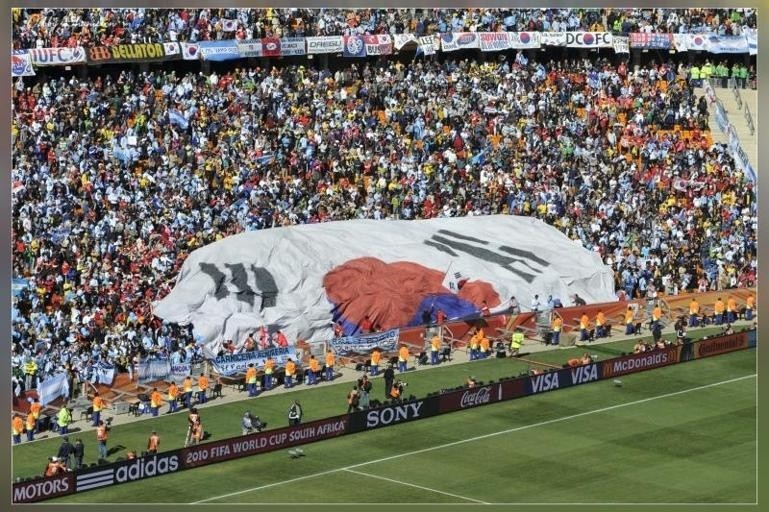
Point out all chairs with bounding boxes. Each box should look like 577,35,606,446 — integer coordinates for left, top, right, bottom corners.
176,383,222,406
68,409,73,422
130,402,140,415
588,330,597,344
645,318,653,330
605,324,613,337
81,406,93,421
634,323,642,336
387,356,400,369
415,352,426,364
659,129,713,147
233,366,326,394
440,348,451,362
359,360,372,374
697,308,747,327
542,329,553,345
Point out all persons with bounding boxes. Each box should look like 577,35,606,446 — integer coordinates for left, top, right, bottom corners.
10,7,757,478
